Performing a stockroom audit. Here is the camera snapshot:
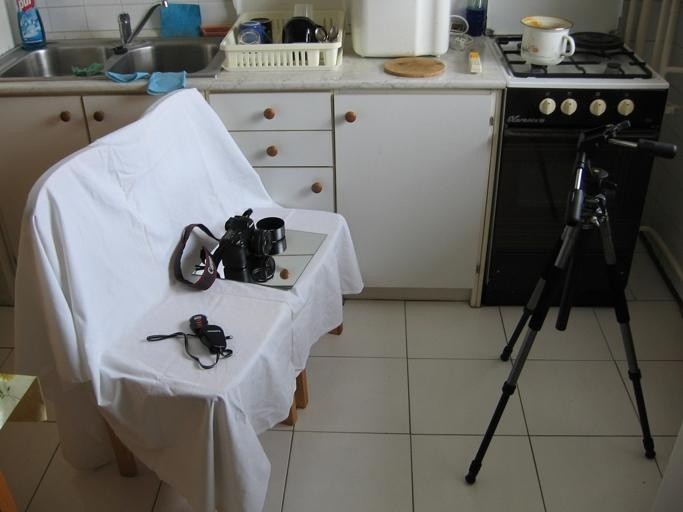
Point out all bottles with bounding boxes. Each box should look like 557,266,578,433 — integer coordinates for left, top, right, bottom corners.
466,0,488,36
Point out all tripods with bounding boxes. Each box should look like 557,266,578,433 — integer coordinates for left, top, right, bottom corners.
465,119,677,485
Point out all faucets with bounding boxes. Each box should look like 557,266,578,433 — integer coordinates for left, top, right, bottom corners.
115,2,170,48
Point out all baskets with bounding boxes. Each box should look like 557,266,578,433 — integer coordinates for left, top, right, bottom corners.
220,11,343,70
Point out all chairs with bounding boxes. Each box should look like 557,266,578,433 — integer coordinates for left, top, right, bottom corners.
24,87,346,512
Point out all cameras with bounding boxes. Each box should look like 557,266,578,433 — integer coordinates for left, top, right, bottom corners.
219,213,275,283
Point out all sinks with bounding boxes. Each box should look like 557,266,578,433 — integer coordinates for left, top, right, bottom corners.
106,41,221,77
0,40,114,80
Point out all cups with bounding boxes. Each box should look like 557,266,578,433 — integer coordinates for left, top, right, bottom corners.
519,13,575,63
238,14,327,46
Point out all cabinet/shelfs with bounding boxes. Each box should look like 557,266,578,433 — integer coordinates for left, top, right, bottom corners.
206,89,336,215
333,90,501,309
0,94,164,264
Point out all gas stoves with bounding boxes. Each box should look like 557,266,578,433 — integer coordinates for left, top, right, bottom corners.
497,34,661,126
